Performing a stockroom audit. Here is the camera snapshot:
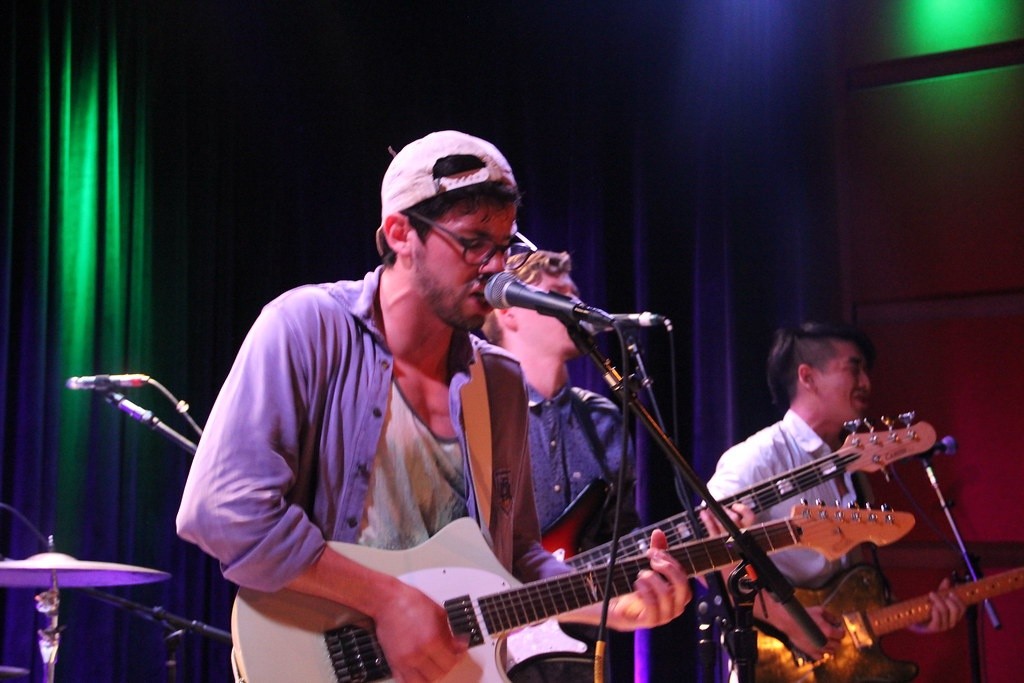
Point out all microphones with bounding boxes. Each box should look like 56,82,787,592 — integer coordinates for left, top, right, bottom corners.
904,436,958,461
579,313,668,335
66,373,150,390
484,271,616,326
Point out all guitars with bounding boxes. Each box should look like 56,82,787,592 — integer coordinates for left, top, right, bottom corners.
749,561,1024,683
231,497,919,683
540,406,937,569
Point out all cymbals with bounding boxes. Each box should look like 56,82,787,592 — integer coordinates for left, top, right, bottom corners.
0,550,174,590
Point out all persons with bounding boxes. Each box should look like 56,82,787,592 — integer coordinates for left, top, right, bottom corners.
691,320,968,682
176,129,691,682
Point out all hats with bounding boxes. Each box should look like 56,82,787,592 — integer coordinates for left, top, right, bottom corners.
374,129,517,257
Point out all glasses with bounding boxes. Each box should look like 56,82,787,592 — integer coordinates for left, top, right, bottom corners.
405,208,537,270
